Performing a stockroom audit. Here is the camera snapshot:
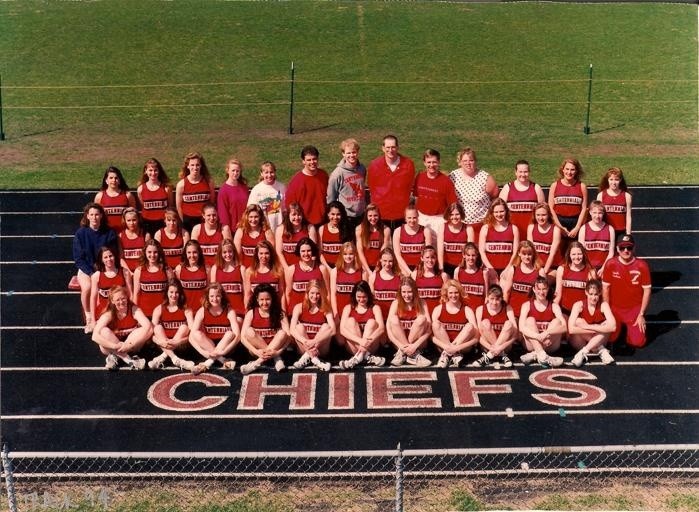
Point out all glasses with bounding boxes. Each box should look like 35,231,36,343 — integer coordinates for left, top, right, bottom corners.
619,245,633,252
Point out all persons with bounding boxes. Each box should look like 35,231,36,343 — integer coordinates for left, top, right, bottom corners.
73,135,651,375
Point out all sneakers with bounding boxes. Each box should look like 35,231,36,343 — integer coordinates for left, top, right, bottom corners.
315,358,331,372
224,357,236,371
176,358,195,370
129,358,147,370
437,346,616,369
406,353,432,367
339,358,357,370
274,355,285,372
367,354,386,366
148,357,164,370
84,319,93,335
105,354,119,372
240,361,261,375
391,349,406,367
191,363,207,375
293,356,310,370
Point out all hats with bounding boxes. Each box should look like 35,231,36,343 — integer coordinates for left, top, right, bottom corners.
616,235,634,246
